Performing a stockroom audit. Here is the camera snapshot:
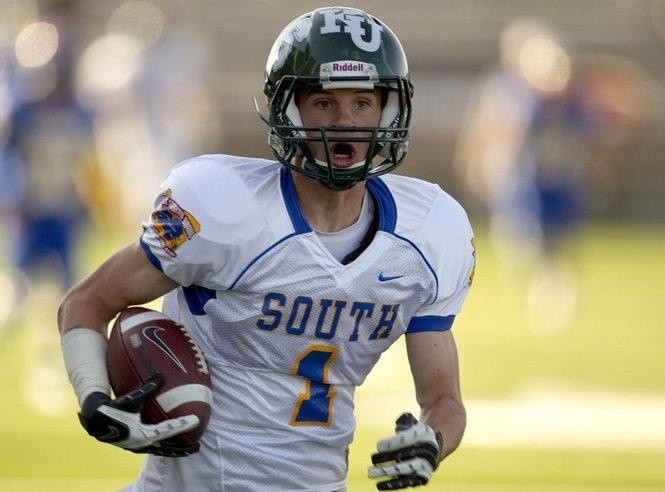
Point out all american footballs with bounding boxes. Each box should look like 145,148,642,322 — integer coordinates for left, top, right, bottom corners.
106,307,212,447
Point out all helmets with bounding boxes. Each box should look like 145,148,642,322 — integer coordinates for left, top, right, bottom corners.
263,6,413,190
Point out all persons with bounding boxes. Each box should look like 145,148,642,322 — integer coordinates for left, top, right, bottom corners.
6,37,93,302
518,52,612,288
57,6,476,491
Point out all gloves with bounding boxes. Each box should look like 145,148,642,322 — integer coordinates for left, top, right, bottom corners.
367,413,444,490
79,374,201,458
